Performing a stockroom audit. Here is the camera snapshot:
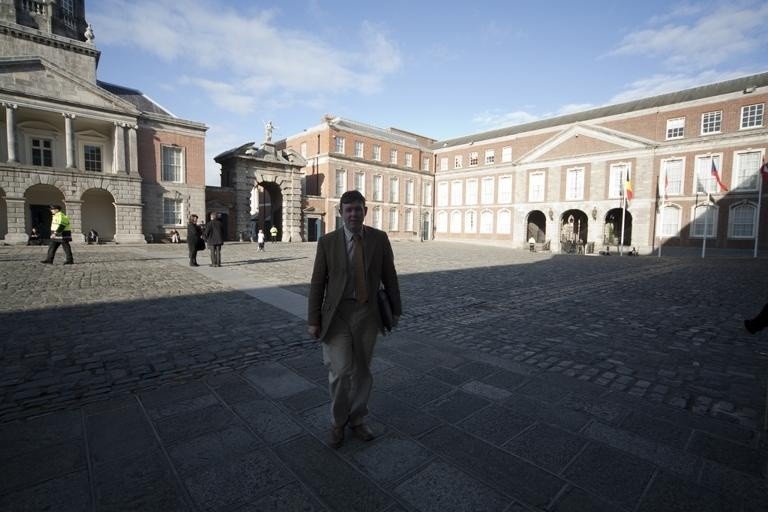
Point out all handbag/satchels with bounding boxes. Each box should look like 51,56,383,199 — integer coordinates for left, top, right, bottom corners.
377,288,393,332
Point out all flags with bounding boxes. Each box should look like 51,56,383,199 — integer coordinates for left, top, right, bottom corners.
665,171,668,199
760,163,768,183
627,169,632,201
711,157,728,192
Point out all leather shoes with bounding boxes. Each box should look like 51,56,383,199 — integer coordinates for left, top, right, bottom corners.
328,426,345,448
348,422,374,441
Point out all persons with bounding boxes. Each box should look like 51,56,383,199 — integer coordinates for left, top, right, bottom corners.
30,227,40,240
200,212,224,267
307,191,403,449
632,247,636,256
739,301,768,337
171,231,180,243
257,229,265,252
40,204,75,265
86,229,97,241
605,246,610,256
187,214,205,266
270,225,278,244
577,240,585,255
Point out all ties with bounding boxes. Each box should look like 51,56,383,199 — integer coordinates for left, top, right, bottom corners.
352,235,368,306
